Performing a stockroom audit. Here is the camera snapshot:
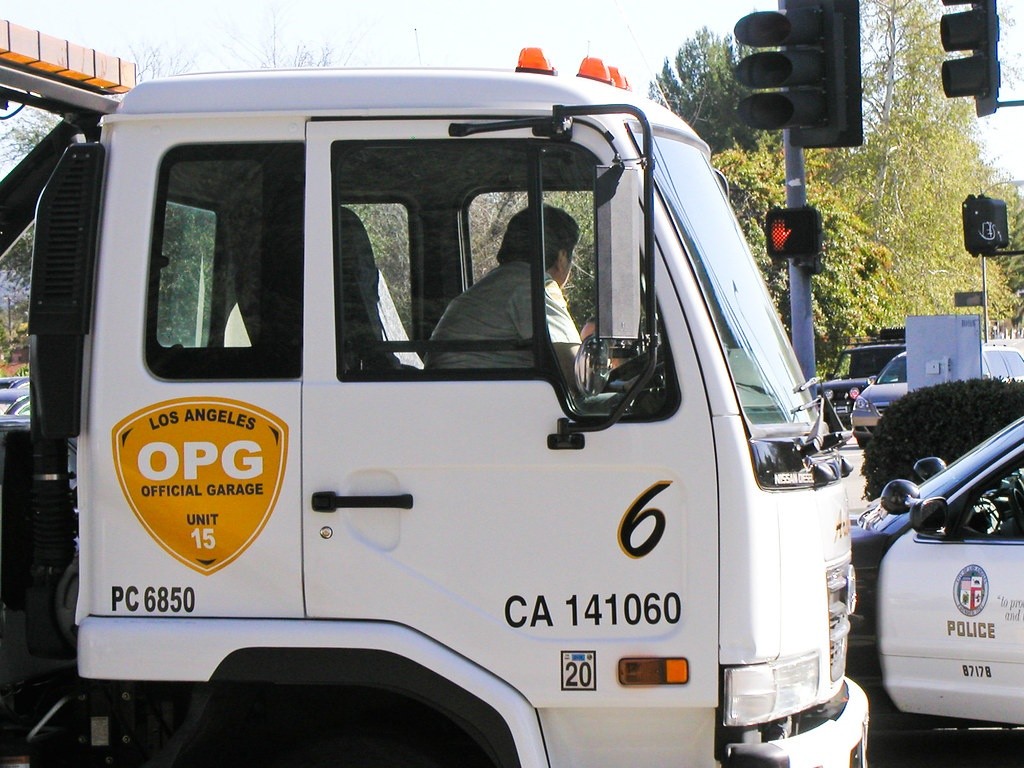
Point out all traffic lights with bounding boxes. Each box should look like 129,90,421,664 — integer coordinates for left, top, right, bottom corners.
961,193,1009,259
733,0,864,149
765,206,823,257
940,0,1002,118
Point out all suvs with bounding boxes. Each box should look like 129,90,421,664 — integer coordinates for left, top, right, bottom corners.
819,327,906,431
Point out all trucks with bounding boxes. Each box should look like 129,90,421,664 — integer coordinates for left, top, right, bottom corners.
0,17,875,768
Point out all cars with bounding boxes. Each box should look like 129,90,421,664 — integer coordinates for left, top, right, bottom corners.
854,416,1024,730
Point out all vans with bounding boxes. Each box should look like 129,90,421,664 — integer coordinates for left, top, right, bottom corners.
850,344,1024,449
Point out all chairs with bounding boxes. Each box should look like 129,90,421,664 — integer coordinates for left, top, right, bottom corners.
226,208,430,370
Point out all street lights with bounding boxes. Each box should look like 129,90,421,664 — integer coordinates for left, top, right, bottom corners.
3,295,12,334
979,180,1024,344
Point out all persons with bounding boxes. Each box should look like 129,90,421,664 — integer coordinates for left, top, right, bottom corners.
423,202,668,417
